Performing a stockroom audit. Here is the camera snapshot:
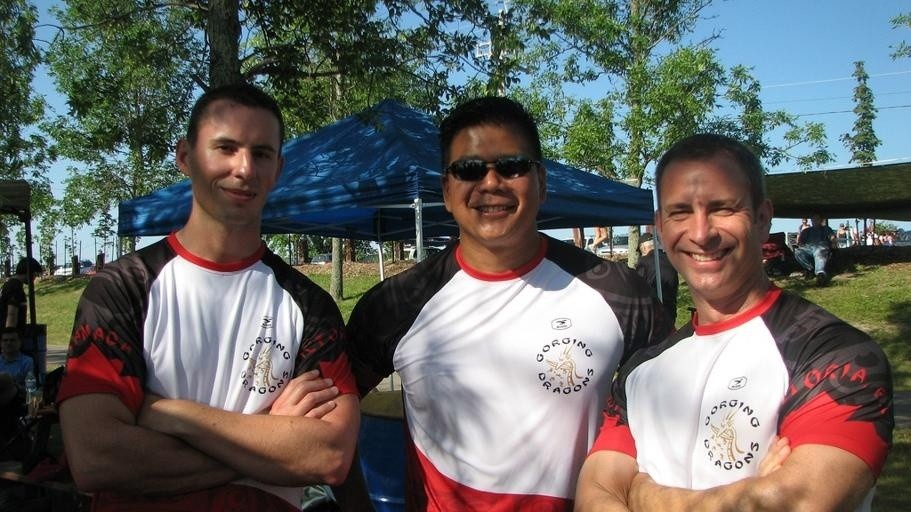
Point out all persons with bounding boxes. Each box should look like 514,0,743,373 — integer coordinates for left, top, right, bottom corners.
344,93,680,512
796,217,810,245
0,255,44,329
570,130,894,512
53,80,363,510
835,222,896,249
0,327,36,465
793,211,838,288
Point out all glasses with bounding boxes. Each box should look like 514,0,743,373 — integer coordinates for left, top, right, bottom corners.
444,156,544,182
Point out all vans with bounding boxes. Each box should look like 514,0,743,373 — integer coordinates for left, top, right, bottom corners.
561,233,629,260
403,244,441,262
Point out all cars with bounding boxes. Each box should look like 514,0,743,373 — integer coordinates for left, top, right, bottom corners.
310,253,333,265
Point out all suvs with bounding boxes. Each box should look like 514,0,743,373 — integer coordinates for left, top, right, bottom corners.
52,260,92,277
